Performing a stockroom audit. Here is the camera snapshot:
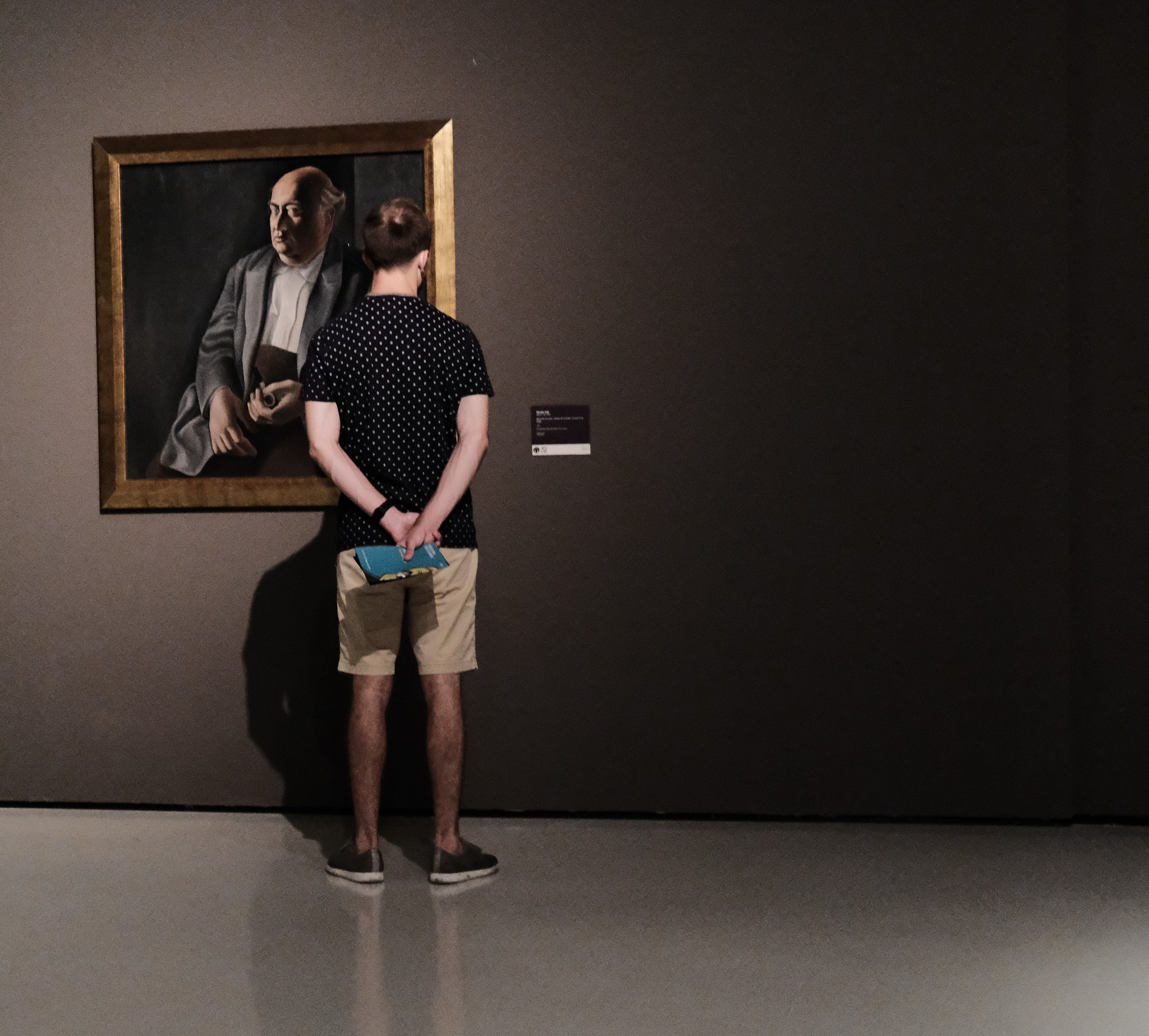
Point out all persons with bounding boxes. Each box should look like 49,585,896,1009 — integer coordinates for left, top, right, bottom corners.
298,197,500,886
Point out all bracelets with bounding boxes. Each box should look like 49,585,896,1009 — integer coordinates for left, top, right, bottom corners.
371,499,394,524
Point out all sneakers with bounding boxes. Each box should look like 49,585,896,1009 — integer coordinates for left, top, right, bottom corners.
324,834,385,884
430,837,499,884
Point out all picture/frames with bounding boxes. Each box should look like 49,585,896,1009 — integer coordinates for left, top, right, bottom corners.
89,118,456,517
